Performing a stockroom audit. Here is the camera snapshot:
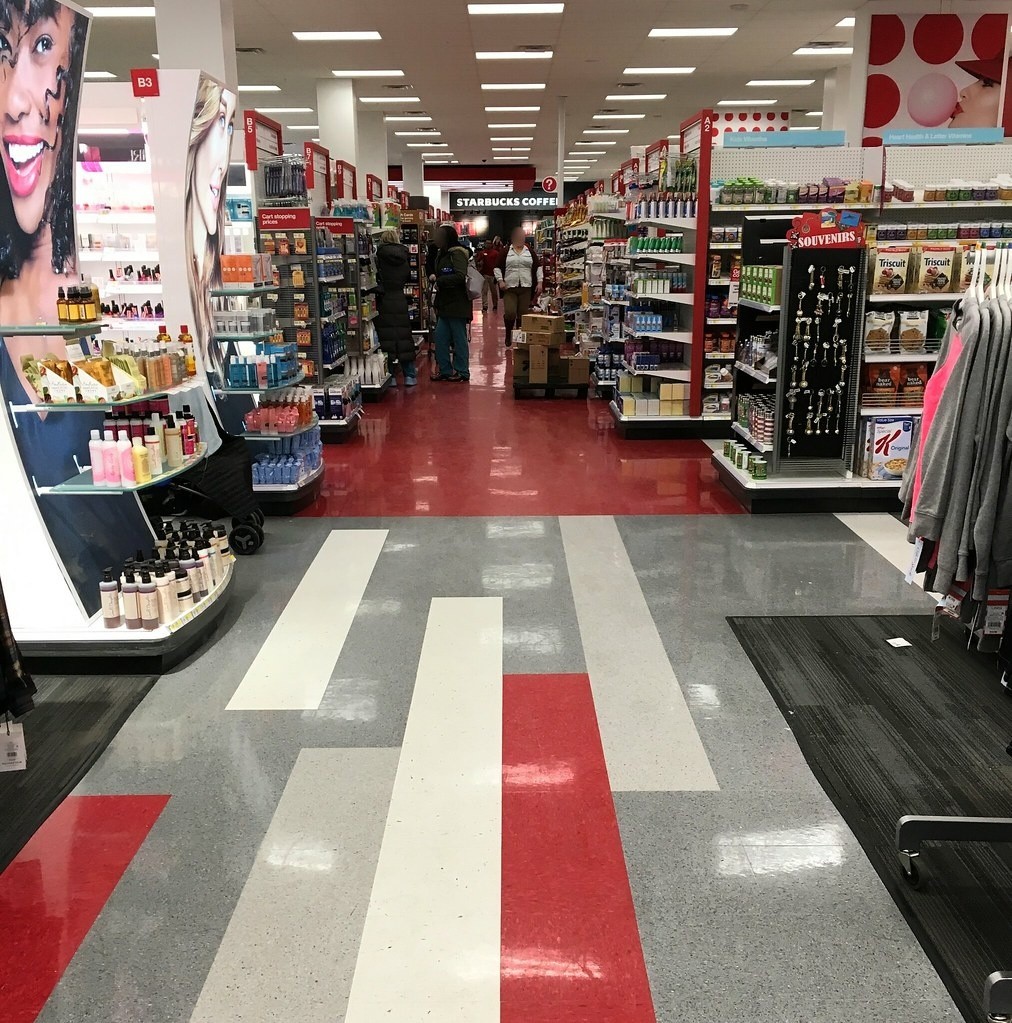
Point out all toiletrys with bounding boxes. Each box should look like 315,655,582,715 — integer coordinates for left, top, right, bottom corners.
98,519,231,632
88,404,202,488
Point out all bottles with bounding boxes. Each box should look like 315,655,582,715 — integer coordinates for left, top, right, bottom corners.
709,176,798,204
229,351,281,389
704,254,741,354
737,393,775,445
344,350,387,385
115,324,196,391
88,395,202,488
636,192,693,219
107,263,161,281
57,273,101,324
100,300,164,319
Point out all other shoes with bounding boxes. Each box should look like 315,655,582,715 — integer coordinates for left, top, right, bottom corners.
405,377,418,385
493,306,497,309
430,372,453,381
448,372,469,381
505,334,512,347
482,308,487,313
389,378,397,386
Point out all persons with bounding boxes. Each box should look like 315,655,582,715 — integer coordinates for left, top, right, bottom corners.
183,73,238,372
492,227,544,348
374,229,419,386
0,0,108,489
425,225,470,383
491,235,504,250
475,240,500,313
947,44,1007,127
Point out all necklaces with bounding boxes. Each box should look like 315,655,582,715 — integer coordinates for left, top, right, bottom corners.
8,254,66,339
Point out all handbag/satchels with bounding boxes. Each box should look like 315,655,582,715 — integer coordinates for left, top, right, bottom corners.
465,266,485,300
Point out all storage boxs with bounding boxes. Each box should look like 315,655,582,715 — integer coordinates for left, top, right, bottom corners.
510,313,592,385
624,306,678,334
624,339,684,372
856,415,915,481
613,375,690,416
633,278,671,294
301,373,360,419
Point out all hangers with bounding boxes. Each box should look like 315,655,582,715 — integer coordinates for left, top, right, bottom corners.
959,247,1012,307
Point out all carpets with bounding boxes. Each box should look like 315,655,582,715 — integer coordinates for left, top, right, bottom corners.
725,613,1012,1023
0,673,161,874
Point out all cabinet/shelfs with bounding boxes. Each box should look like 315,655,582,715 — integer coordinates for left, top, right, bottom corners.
712,214,1012,514
253,207,363,443
534,200,641,397
609,110,1012,439
0,206,325,674
338,208,436,404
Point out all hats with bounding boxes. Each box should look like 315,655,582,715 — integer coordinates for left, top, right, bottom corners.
955,48,1004,84
